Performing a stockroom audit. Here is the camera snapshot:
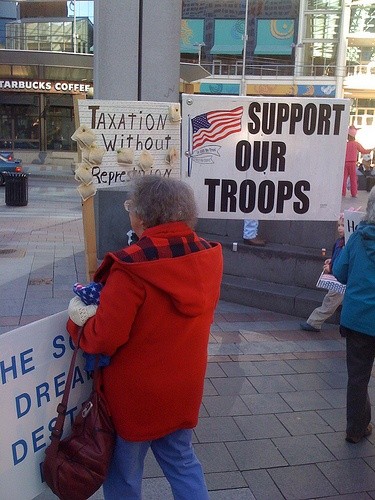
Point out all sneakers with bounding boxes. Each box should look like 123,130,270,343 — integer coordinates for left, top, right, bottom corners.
300,321,321,332
346,422,372,444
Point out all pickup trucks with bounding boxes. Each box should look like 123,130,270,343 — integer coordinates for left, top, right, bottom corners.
0,152,23,185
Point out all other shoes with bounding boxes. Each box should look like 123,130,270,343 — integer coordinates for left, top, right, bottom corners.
244,237,267,246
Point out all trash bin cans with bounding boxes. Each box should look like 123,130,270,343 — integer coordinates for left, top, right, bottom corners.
2,170,29,207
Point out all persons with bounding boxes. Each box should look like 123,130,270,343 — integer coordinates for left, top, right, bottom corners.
66,177,224,500
299,216,346,332
342,132,375,195
243,218,268,247
333,188,375,443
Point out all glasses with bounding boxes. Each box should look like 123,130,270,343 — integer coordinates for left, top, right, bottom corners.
123,199,135,212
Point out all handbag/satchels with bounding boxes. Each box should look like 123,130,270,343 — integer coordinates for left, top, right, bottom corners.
42,316,114,500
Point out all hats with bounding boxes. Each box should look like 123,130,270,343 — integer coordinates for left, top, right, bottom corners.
363,154,372,161
